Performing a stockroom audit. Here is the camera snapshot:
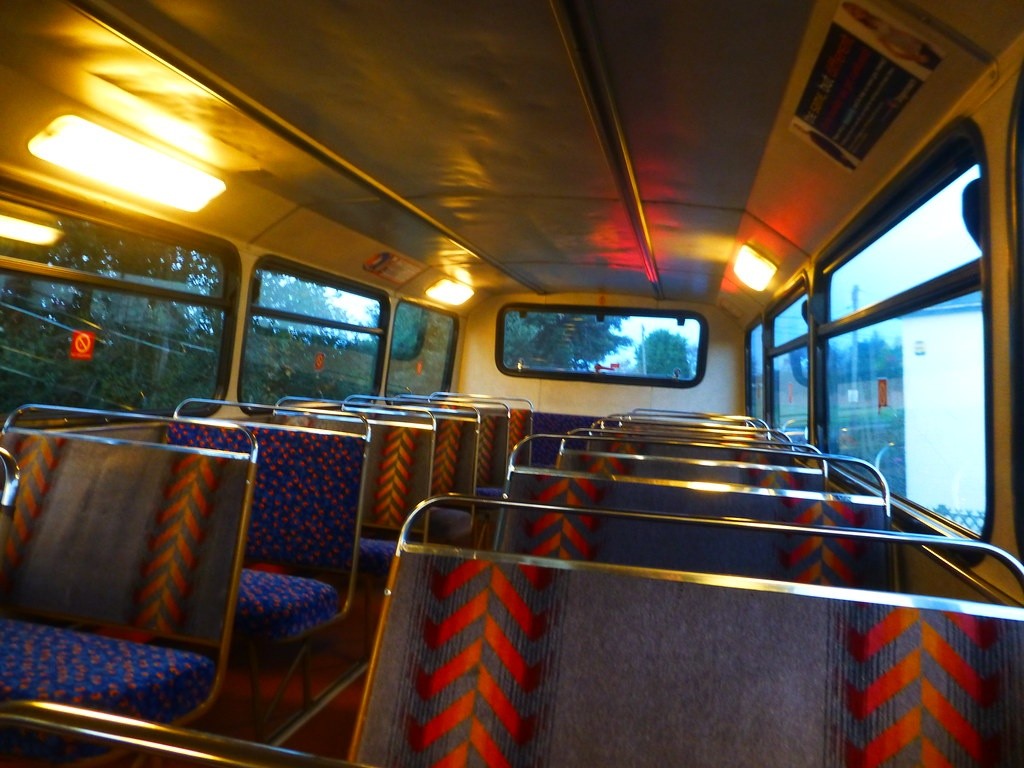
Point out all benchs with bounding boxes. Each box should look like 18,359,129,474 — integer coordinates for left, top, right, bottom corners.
0,391,1024,768
